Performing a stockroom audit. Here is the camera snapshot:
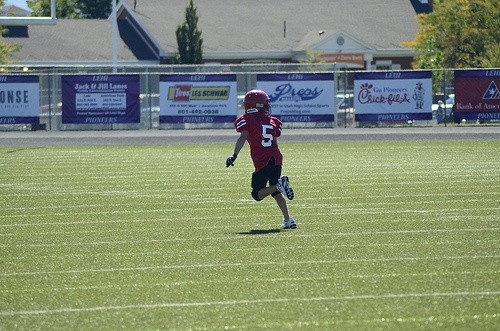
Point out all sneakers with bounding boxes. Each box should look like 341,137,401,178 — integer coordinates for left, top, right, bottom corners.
277,176,294,200
280,218,297,229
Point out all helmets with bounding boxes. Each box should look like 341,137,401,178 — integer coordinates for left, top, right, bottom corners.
242,89,272,116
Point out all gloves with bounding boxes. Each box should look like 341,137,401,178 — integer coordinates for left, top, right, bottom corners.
226,154,237,168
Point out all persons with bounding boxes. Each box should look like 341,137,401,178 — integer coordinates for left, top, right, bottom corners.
225,89,297,229
